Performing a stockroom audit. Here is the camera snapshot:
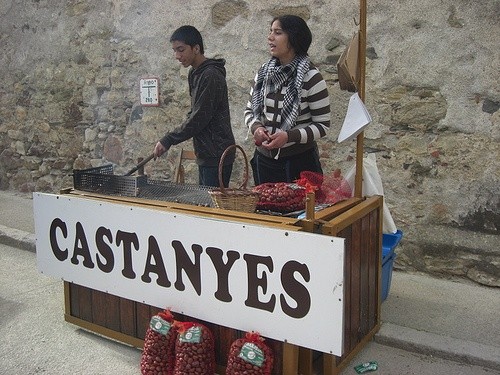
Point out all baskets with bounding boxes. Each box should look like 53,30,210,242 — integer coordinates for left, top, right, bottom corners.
208,145,259,214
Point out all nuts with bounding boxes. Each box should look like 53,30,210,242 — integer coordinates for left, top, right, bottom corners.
251,182,306,212
140,315,272,375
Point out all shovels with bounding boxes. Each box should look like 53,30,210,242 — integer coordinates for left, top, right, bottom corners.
124,151,162,176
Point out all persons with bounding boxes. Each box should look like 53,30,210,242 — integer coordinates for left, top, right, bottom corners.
147,23,237,189
243,13,333,190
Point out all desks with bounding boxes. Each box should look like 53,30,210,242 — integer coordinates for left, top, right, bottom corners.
31,174,384,375
